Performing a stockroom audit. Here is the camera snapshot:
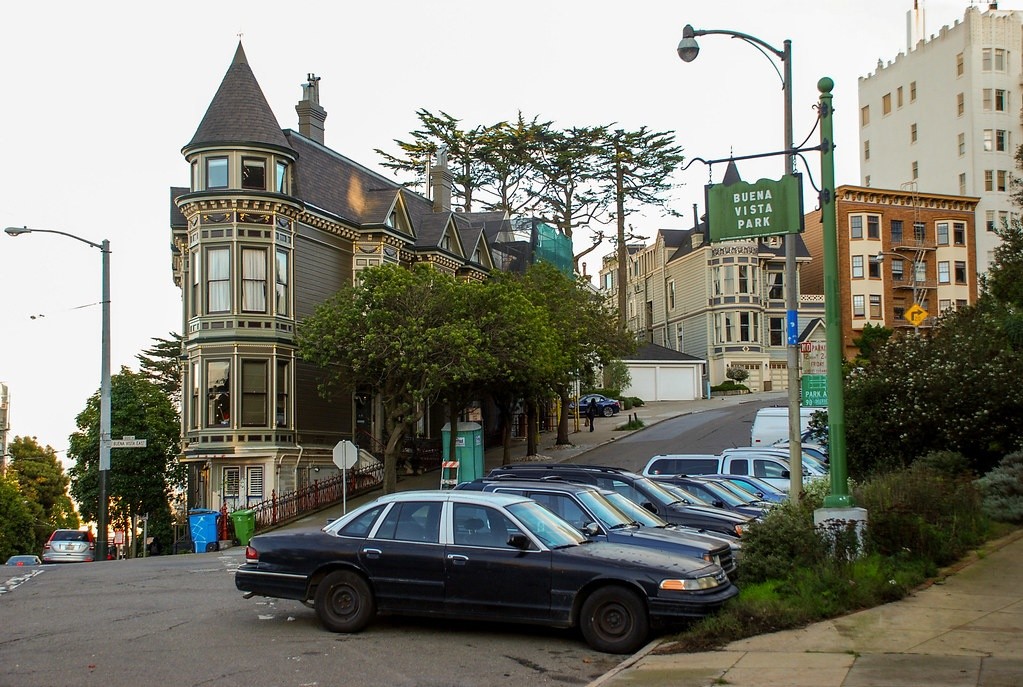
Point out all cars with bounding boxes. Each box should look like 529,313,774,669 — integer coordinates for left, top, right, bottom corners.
767,442,832,466
569,394,619,417
721,444,831,477
3,555,43,567
779,424,832,451
235,489,742,653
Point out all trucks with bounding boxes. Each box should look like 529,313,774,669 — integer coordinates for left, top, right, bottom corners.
645,450,835,514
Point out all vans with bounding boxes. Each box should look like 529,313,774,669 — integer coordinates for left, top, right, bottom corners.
750,406,832,450
41,528,96,563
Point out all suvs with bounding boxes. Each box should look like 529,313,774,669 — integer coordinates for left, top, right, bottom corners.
648,471,791,520
452,476,745,587
488,462,759,567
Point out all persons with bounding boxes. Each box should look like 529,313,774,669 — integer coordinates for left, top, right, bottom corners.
584,397,599,432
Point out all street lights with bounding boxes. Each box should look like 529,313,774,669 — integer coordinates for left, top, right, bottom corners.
4,226,113,564
675,25,804,513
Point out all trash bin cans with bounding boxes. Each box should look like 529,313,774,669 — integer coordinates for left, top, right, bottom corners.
188,509,221,555
230,510,258,548
